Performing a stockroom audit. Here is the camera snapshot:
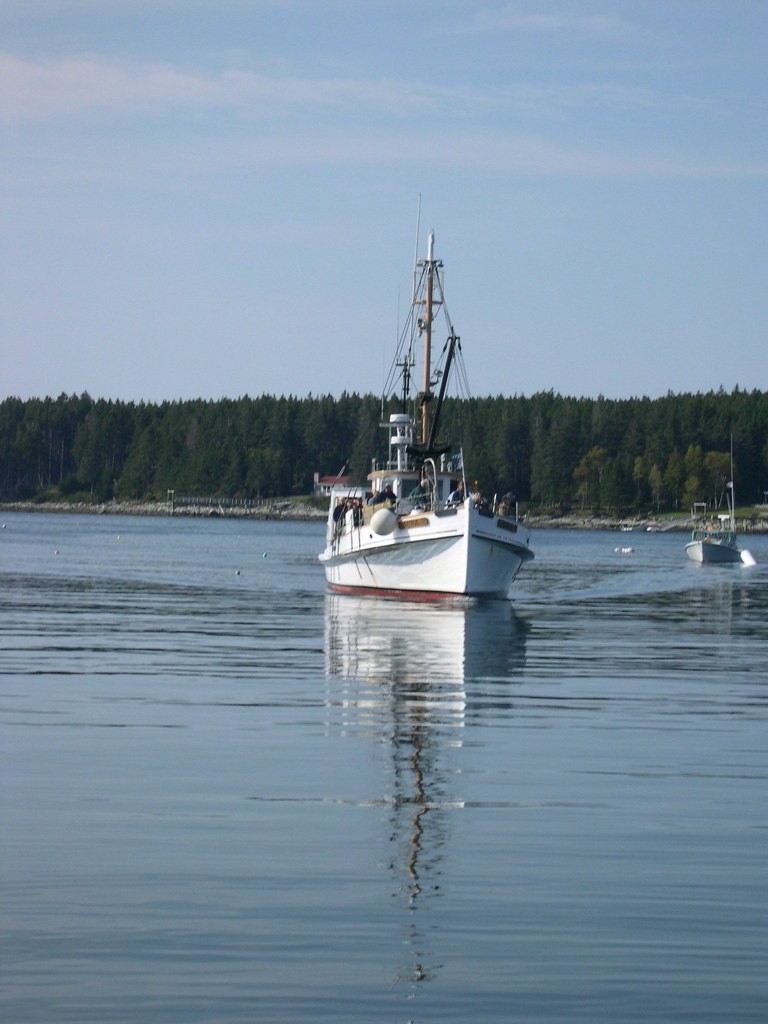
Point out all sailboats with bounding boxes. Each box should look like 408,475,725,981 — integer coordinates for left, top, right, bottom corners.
685,431,743,563
318,191,535,604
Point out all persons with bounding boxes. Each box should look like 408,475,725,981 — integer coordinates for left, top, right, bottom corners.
366,483,400,509
333,497,364,529
408,480,430,512
444,480,516,519
707,522,715,531
